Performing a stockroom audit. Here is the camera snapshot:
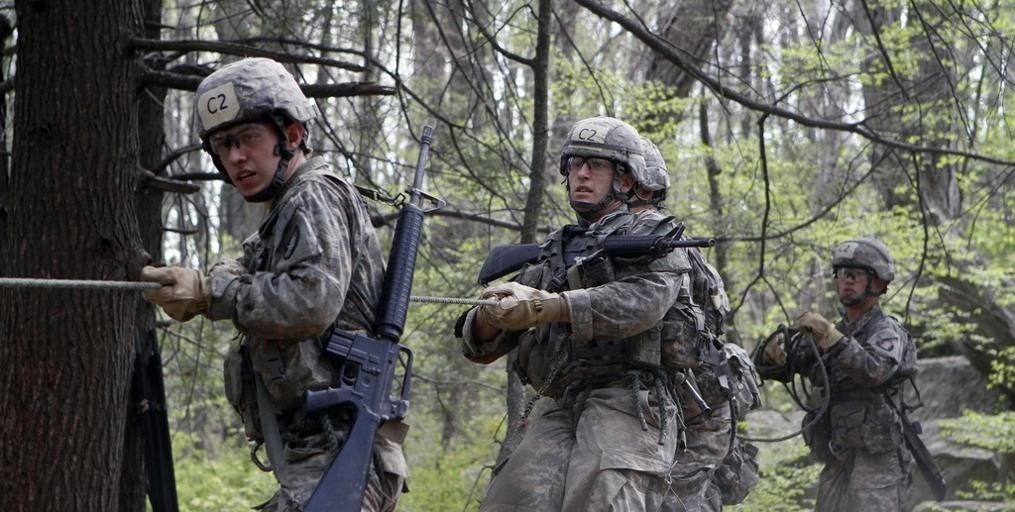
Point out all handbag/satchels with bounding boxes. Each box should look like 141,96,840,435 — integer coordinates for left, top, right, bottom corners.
713,444,759,505
722,343,762,415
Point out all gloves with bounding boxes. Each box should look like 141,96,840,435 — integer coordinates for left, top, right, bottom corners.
793,312,844,352
761,333,787,364
141,266,212,322
480,282,563,331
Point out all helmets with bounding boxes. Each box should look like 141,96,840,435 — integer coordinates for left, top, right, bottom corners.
195,57,317,136
560,116,670,201
832,237,894,281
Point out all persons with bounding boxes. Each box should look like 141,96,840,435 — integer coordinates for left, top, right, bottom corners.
138,54,411,512
625,138,764,512
753,237,920,512
453,115,713,512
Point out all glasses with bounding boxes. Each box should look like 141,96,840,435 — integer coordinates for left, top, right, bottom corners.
566,157,614,174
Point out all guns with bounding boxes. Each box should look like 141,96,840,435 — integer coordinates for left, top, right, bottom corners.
478,227,713,287
298,124,446,510
